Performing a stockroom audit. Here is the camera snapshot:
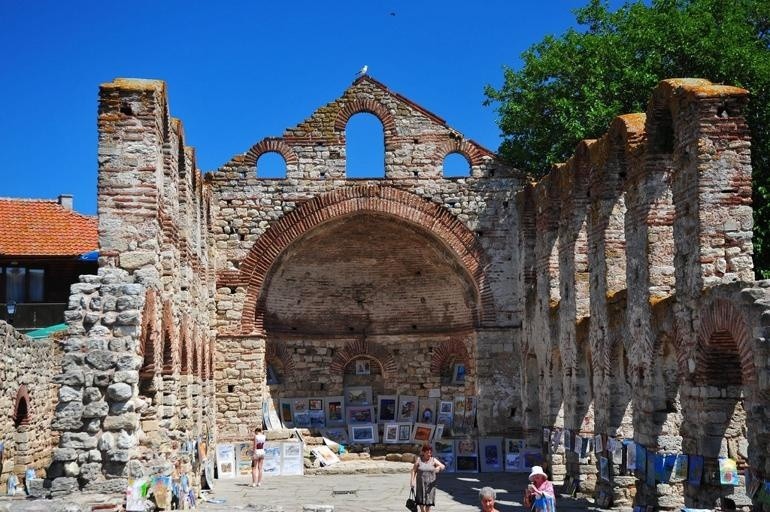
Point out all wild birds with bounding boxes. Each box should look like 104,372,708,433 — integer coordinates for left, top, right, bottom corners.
353,65,368,77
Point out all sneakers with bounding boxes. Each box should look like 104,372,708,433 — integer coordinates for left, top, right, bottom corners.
248,483,260,487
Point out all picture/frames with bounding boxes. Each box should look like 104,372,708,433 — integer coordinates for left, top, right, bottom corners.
267,365,279,384
452,364,465,384
503,437,527,473
478,436,503,472
280,386,477,474
520,448,545,472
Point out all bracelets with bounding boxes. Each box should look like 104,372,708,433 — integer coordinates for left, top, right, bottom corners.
437,466,441,471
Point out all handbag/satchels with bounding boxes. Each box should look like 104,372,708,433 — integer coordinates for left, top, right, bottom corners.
406,499,418,512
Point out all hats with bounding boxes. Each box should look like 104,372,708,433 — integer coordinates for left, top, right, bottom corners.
528,465,548,481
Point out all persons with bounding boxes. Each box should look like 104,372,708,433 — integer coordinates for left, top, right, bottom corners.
422,408,431,423
458,439,476,454
328,403,339,419
477,486,500,512
411,443,446,512
352,411,368,423
246,427,266,487
523,465,556,512
